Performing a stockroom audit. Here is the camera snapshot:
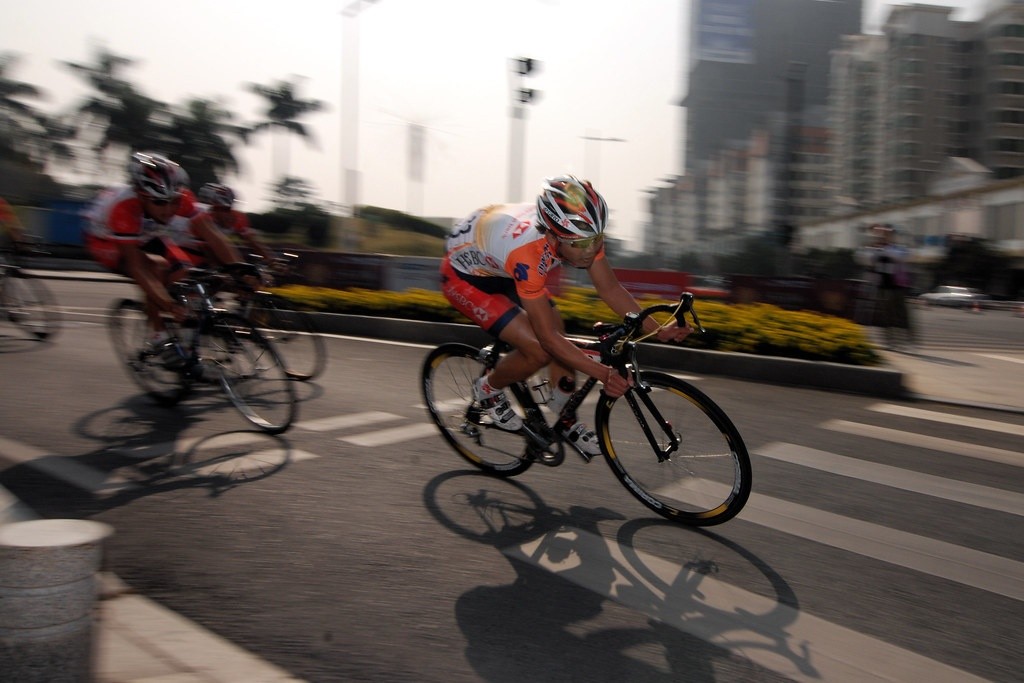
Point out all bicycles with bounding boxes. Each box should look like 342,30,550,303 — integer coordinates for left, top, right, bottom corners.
0,260,61,342
105,252,328,436
420,288,752,527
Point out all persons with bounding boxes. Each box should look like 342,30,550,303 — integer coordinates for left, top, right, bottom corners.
0,198,29,319
91,152,287,356
441,174,694,455
859,224,915,346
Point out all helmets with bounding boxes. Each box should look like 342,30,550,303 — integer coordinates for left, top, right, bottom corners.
197,182,234,209
129,152,184,200
536,173,609,239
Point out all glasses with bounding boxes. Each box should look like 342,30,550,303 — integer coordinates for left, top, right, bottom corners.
557,234,606,248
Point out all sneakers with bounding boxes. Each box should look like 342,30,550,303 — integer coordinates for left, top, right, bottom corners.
565,422,602,455
474,379,523,430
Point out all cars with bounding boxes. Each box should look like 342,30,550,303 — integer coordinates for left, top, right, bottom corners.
917,286,990,310
